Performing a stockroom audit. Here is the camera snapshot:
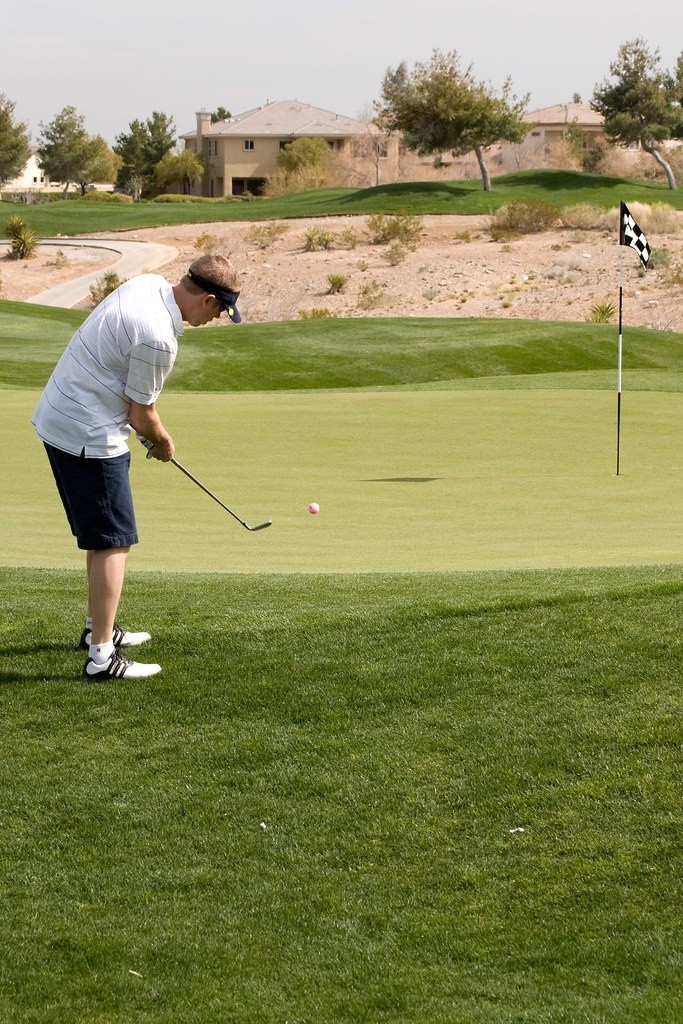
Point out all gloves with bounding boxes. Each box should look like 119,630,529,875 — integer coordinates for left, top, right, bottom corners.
135,433,155,459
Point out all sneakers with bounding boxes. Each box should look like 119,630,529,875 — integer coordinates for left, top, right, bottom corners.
84,648,162,680
78,620,152,649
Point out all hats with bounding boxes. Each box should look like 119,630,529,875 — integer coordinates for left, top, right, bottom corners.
186,269,242,324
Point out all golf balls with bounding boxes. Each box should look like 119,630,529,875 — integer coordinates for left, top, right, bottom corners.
309,502,321,513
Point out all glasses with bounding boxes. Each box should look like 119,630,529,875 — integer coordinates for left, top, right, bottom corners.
215,299,225,313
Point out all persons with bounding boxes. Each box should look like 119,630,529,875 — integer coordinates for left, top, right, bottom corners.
30,254,242,677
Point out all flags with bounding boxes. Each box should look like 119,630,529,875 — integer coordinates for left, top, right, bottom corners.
618,199,651,273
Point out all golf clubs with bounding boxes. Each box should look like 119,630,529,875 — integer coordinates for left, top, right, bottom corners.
125,417,272,532
